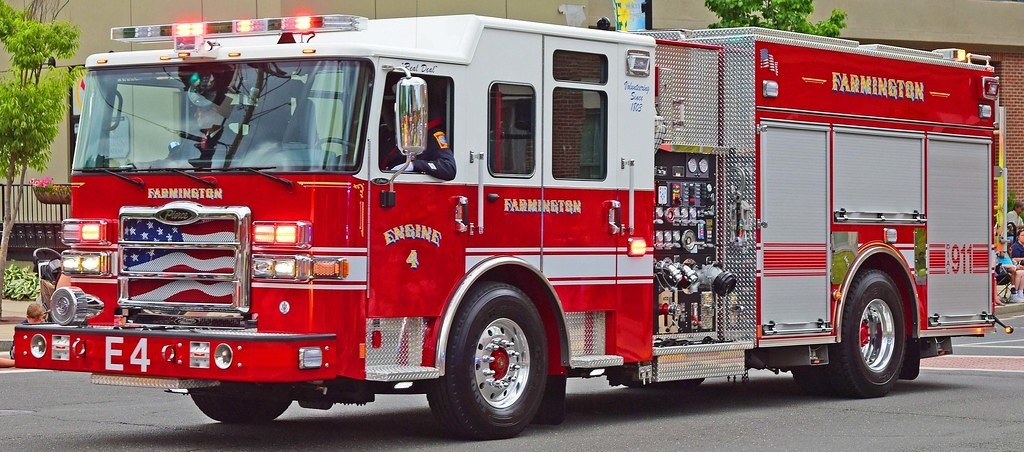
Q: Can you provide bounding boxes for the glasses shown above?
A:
[1019,229,1024,232]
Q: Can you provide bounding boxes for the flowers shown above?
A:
[27,177,71,196]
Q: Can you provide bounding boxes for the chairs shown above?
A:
[995,260,1022,303]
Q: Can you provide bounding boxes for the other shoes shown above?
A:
[1018,290,1024,299]
[995,301,1005,306]
[1009,294,1024,303]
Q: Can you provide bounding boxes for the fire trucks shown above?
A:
[7,12,1015,439]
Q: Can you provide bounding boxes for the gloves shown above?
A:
[390,162,414,171]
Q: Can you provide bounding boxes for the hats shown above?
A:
[368,72,403,100]
[1014,202,1024,210]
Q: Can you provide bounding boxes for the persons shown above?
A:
[995,202,1024,306]
[165,93,249,163]
[0,358,14,367]
[379,84,457,181]
[10,303,44,359]
